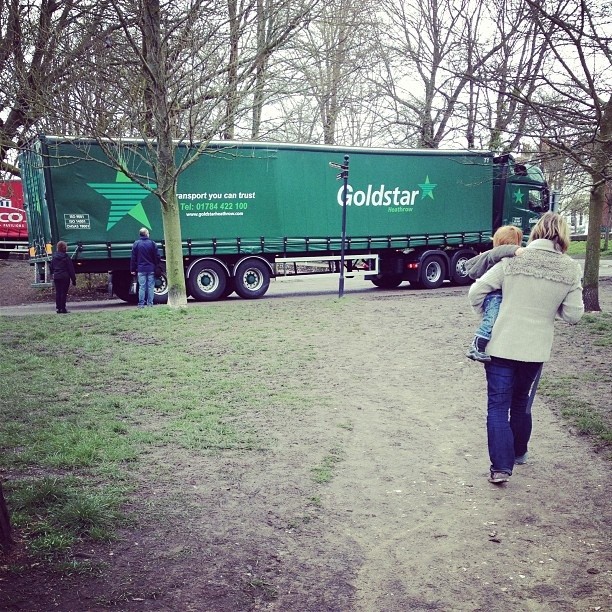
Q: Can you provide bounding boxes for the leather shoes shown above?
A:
[56,309,60,313]
[60,309,70,313]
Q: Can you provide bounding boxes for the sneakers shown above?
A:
[488,471,510,483]
[466,337,491,362]
[514,457,527,465]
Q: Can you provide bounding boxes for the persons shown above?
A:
[465,225,526,362]
[468,211,584,484]
[52,241,76,314]
[130,227,162,308]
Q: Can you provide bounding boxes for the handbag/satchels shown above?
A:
[152,241,162,278]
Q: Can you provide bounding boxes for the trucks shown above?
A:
[17,135,553,299]
